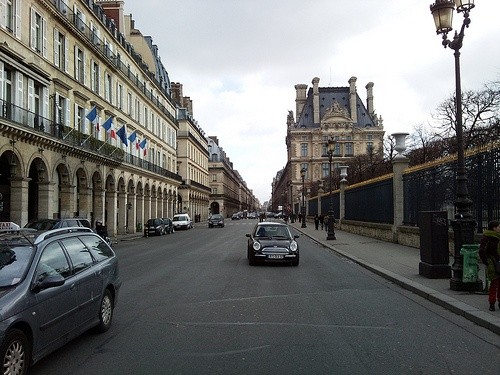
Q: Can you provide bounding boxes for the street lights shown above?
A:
[299,168,308,228]
[290,182,295,223]
[429,0,484,292]
[325,137,337,241]
[281,190,287,219]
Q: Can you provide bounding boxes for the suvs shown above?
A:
[172,213,191,229]
[0,225,122,375]
[23,219,92,234]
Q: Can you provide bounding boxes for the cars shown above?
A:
[0,221,37,241]
[144,218,166,236]
[275,212,285,219]
[207,214,224,228]
[162,218,174,234]
[245,222,300,266]
[231,211,274,220]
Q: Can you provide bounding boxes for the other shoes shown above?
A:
[489,302,496,311]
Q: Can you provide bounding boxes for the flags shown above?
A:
[102,116,116,139]
[86,107,99,131]
[139,139,146,156]
[128,131,139,149]
[116,125,128,147]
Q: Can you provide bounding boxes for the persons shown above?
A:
[320,213,328,231]
[260,213,266,222]
[314,214,320,230]
[199,214,201,222]
[479,220,500,312]
[286,210,302,223]
[96,221,104,238]
